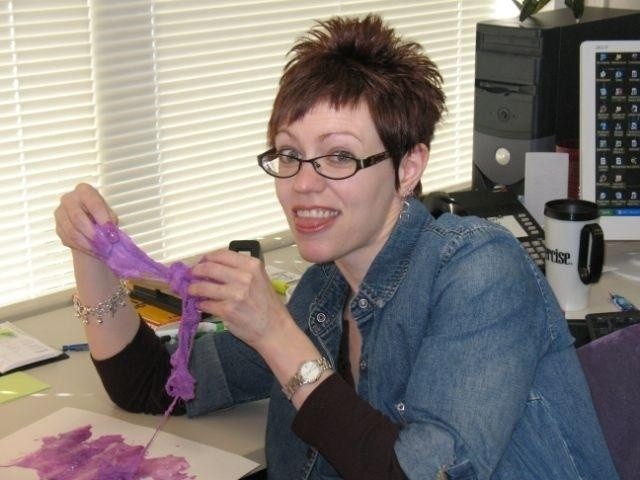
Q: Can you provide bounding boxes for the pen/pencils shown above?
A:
[62,344,89,351]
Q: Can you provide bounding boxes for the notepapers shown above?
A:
[0,370,52,405]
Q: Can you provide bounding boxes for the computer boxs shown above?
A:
[472,5,640,198]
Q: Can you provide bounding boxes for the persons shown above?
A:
[51,10,617,478]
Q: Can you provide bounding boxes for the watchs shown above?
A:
[281,356,332,401]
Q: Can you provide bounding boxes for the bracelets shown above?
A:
[71,281,131,327]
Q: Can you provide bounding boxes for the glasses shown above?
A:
[256,146,388,181]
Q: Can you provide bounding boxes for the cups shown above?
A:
[542,198,605,312]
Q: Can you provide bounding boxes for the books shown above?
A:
[0,321,69,378]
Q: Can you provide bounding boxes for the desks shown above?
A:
[0,182,640,480]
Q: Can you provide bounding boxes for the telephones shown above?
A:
[422,189,546,277]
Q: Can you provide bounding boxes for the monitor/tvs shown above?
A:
[579,39,640,240]
